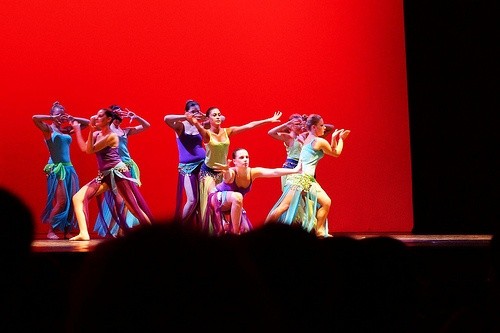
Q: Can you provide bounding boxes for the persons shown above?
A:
[33,102,90,240]
[185,107,283,236]
[0,189,500,333]
[69,110,151,241]
[93,105,150,240]
[264,114,351,237]
[164,100,225,229]
[202,148,302,234]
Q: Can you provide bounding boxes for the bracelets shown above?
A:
[339,136,344,140]
